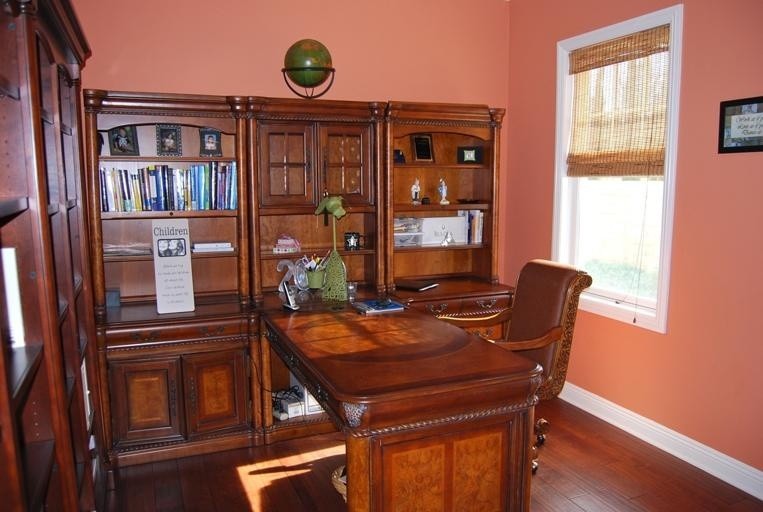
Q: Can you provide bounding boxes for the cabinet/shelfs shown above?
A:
[246,96,387,204]
[246,205,387,307]
[387,100,506,291]
[392,291,514,342]
[0,1,115,512]
[83,89,245,314]
[93,316,250,465]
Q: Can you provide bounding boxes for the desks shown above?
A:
[258,302,544,511]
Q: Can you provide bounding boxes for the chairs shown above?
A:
[433,257,593,472]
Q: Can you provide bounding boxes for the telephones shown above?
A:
[282,281,300,310]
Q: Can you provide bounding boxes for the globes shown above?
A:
[282,39,336,99]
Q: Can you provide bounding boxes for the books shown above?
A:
[100,161,238,212]
[458,209,484,244]
[190,242,235,253]
[353,299,405,313]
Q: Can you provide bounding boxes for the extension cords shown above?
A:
[272,405,289,421]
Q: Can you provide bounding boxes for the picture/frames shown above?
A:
[199,127,222,157]
[154,124,182,157]
[107,124,139,157]
[410,133,434,163]
[716,95,763,154]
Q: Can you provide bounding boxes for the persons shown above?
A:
[160,133,175,151]
[438,179,447,202]
[204,136,217,150]
[169,240,183,256]
[158,240,172,257]
[410,180,420,204]
[114,127,134,153]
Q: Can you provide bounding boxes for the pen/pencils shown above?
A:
[303,248,332,271]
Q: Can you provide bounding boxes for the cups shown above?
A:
[306,270,326,290]
[347,281,358,302]
[344,231,366,250]
